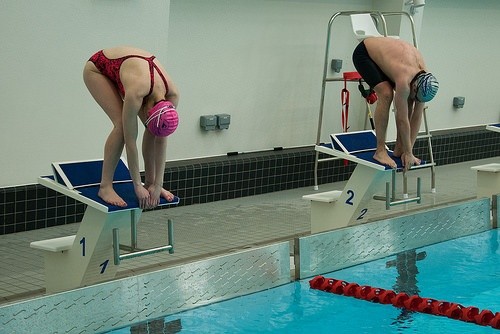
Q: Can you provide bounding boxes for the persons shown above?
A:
[83,45,179,210]
[352,36,439,175]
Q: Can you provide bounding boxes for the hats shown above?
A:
[146,101,179,137]
[414,72,439,102]
